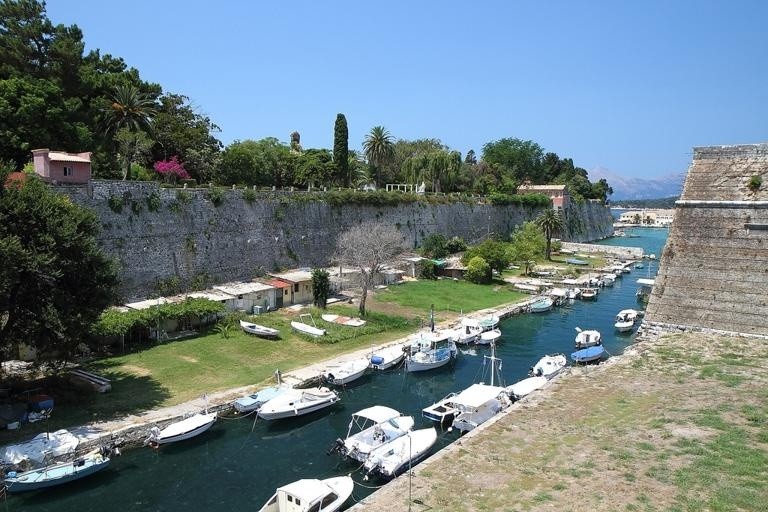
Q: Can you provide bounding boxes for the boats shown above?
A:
[549,288,570,308]
[0,438,125,496]
[406,346,452,372]
[614,317,634,333]
[420,383,513,432]
[142,410,218,446]
[587,259,644,287]
[616,308,638,323]
[529,297,553,313]
[326,415,415,463]
[454,324,484,344]
[239,320,280,338]
[568,287,584,300]
[527,352,568,380]
[320,357,369,385]
[364,426,438,478]
[368,343,405,370]
[233,383,296,413]
[474,328,502,344]
[257,385,341,421]
[636,278,656,288]
[290,320,327,338]
[581,287,597,301]
[570,344,605,363]
[574,329,601,348]
[504,375,548,400]
[478,314,500,332]
[261,472,355,509]
[321,313,366,328]
